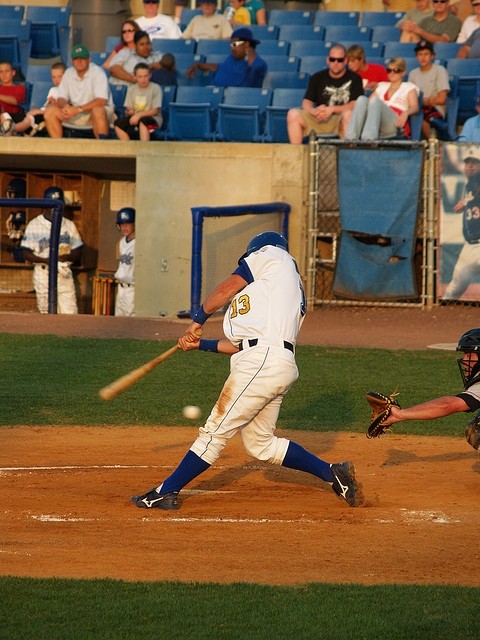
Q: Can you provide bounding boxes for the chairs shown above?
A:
[0,5,127,120]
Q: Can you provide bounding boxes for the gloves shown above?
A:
[57,261,72,278]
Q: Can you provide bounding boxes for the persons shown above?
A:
[114,63,163,141]
[407,40,451,141]
[457,0,480,43]
[131,231,363,510]
[113,208,135,318]
[224,0,251,26]
[345,56,421,141]
[227,0,267,26]
[376,328,480,451]
[180,0,234,40]
[0,62,67,137]
[187,28,267,87]
[402,1,461,43]
[20,186,84,314]
[43,44,115,139]
[0,62,26,137]
[457,98,480,143]
[443,145,480,300]
[108,30,163,88]
[394,0,457,43]
[134,0,183,39]
[346,45,390,90]
[150,54,177,84]
[100,20,141,68]
[287,44,365,147]
[455,27,480,59]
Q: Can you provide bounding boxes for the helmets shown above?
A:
[44,186,64,199]
[456,328,480,391]
[5,178,26,198]
[12,211,26,224]
[238,231,288,265]
[116,207,135,224]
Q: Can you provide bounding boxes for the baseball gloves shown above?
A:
[365,392,401,438]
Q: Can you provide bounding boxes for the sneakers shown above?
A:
[131,487,179,510]
[330,461,356,508]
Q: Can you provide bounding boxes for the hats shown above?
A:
[231,27,260,44]
[414,40,433,52]
[462,145,480,162]
[71,44,89,59]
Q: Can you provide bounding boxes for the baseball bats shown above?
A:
[98,327,202,403]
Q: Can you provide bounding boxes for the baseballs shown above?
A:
[183,405,200,421]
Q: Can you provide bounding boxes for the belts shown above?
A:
[239,338,293,353]
[121,282,130,287]
[469,239,480,244]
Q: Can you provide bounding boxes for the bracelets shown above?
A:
[193,305,212,326]
[198,339,220,354]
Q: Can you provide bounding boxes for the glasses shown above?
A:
[387,67,403,73]
[473,3,480,6]
[145,1,157,3]
[203,1,215,4]
[433,1,446,3]
[122,29,135,33]
[230,41,245,47]
[329,57,344,62]
